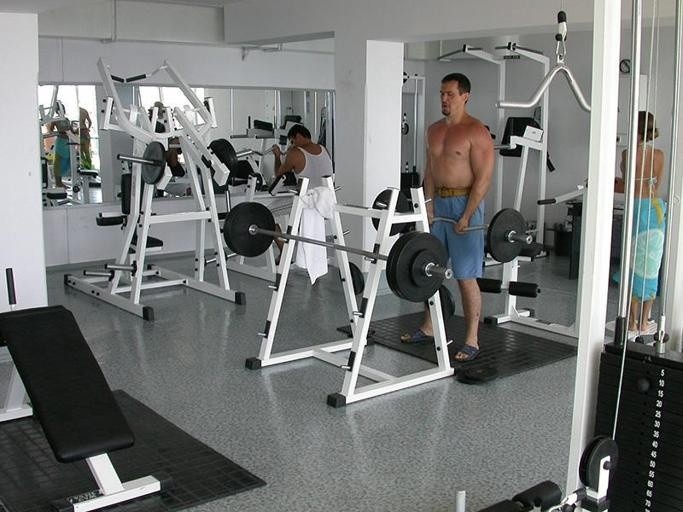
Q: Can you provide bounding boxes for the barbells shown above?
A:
[224,202,454,302]
[372,189,532,262]
[116,138,254,184]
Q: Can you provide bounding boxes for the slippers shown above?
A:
[455,344,480,362]
[402,329,434,343]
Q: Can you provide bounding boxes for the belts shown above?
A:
[436,187,470,197]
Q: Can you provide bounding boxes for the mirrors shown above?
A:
[400,93,418,191]
[41,80,335,210]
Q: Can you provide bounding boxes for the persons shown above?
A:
[614,111,665,332]
[79,107,92,168]
[400,72,494,361]
[48,102,73,190]
[43,120,57,155]
[147,102,185,178]
[272,124,335,265]
